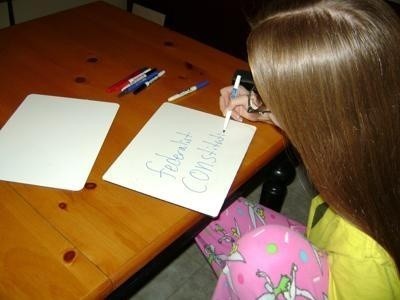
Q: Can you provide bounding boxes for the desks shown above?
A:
[0,0,315,299]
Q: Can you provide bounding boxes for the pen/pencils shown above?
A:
[106,66,167,98]
[167,79,209,102]
[222,75,242,133]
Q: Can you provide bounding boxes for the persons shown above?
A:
[195,0,400,300]
